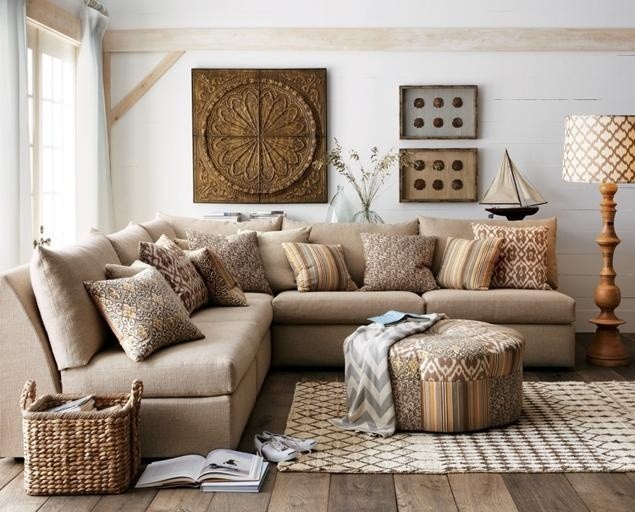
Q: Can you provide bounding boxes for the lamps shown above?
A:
[562,114,635,367]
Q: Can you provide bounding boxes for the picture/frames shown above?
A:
[399,85,478,140]
[399,148,478,203]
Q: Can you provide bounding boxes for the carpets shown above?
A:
[276,379,635,474]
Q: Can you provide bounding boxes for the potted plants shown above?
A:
[312,137,422,224]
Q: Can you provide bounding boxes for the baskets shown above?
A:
[20,377,144,498]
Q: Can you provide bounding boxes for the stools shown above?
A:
[357,318,526,434]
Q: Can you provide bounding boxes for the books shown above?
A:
[367,308,430,327]
[134,448,265,485]
[199,459,272,494]
[48,394,122,414]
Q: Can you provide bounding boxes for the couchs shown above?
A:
[1,265,574,464]
